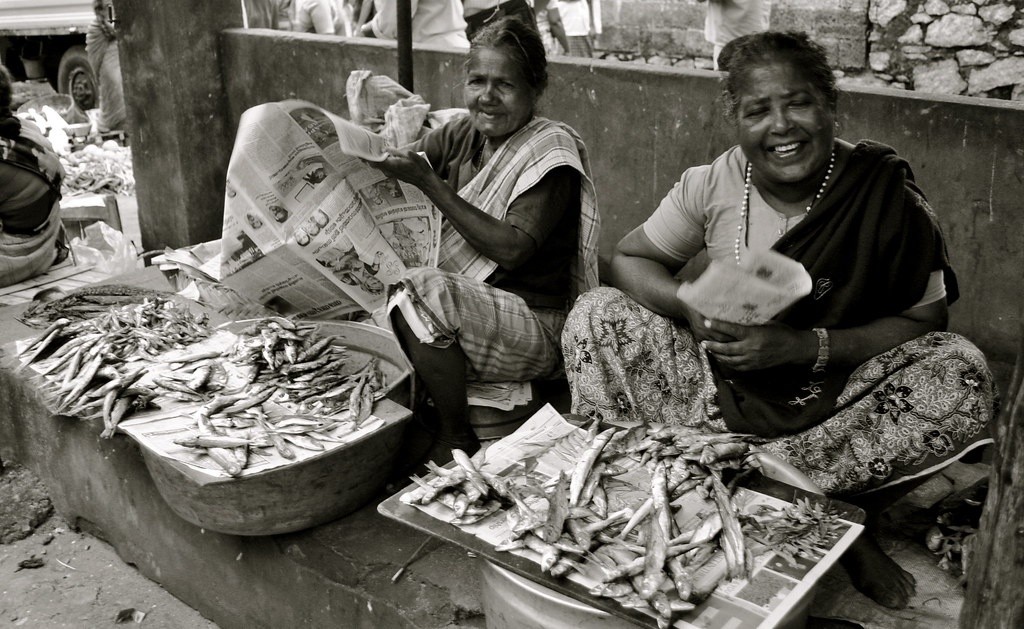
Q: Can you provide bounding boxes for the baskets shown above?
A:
[17,93,75,123]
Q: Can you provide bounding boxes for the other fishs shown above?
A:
[410,407,784,629]
[52,142,136,198]
[17,298,389,477]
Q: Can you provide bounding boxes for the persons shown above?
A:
[367,14,601,472]
[246,213,263,229]
[316,234,385,295]
[294,211,329,246]
[268,205,288,223]
[360,178,403,205]
[86,0,126,132]
[0,61,67,287]
[561,32,1000,607]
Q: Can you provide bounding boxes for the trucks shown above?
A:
[0,2,101,113]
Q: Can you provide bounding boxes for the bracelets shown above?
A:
[812,328,831,373]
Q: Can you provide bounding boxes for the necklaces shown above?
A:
[734,147,835,267]
[476,140,488,172]
